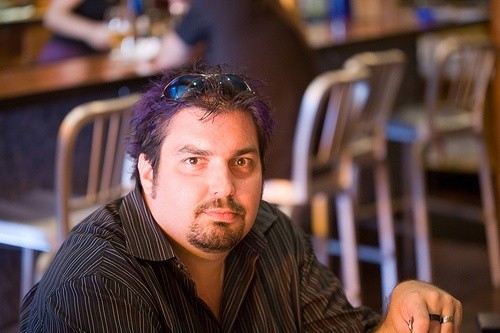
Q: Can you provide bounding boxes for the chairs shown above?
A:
[0,92,145,312]
[260,47,406,313]
[368,34,500,287]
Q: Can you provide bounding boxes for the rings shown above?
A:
[442,315,454,323]
[429,313,443,324]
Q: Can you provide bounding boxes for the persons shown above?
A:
[18,58,463,333]
[34,0,329,181]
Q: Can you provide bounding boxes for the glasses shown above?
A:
[159,73,256,104]
